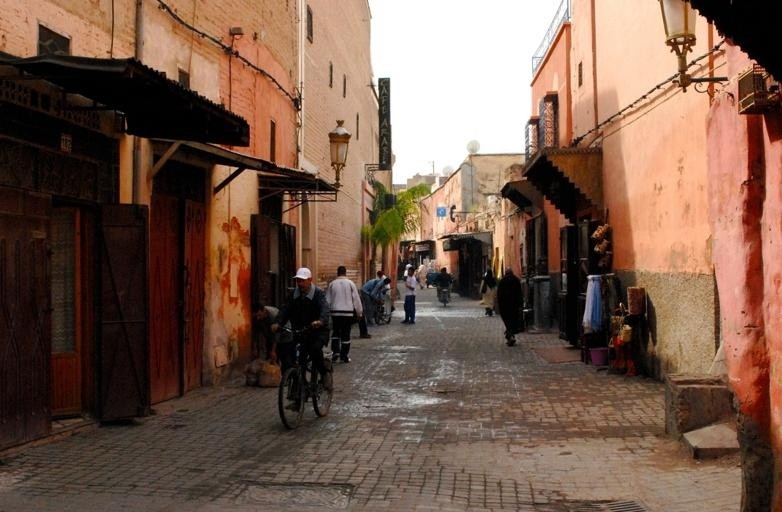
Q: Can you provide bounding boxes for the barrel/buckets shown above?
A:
[590,347,608,366]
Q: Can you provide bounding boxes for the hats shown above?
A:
[292,268,312,280]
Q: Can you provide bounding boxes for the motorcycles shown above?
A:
[432,279,453,307]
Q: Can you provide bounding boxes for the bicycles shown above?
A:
[275,324,334,429]
[373,286,392,326]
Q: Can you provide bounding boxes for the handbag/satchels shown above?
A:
[244,359,282,387]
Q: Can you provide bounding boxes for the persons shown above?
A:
[479,268,497,317]
[271,268,330,410]
[377,271,391,316]
[434,268,452,300]
[401,268,417,324]
[325,266,364,363]
[403,260,412,277]
[251,301,297,386]
[361,278,391,327]
[497,267,525,345]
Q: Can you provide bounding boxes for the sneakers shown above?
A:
[321,375,331,391]
[360,333,371,338]
[400,320,416,324]
[506,335,517,346]
[332,353,351,363]
[285,402,305,412]
